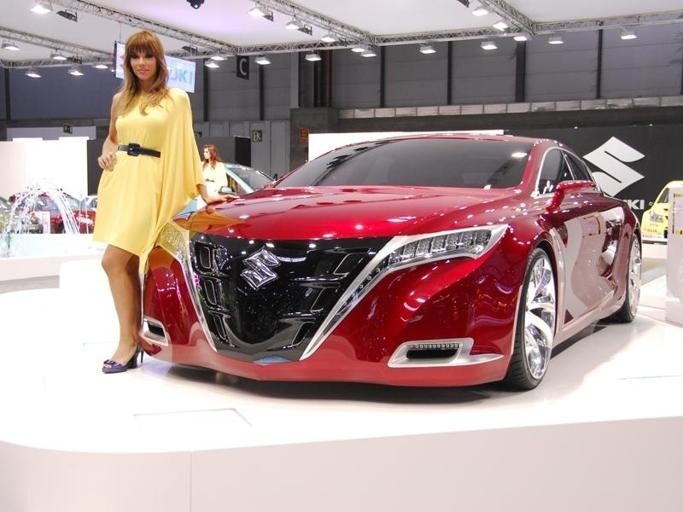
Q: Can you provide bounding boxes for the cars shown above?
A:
[637,179,683,243]
[0,187,99,233]
[221,161,277,200]
[131,126,645,396]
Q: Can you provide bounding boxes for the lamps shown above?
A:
[418,45,437,55]
[28,2,52,15]
[620,28,638,41]
[350,45,377,59]
[492,21,509,33]
[547,34,565,46]
[285,20,313,39]
[2,42,21,54]
[56,9,78,24]
[92,63,109,72]
[68,67,84,77]
[49,54,69,62]
[304,53,322,62]
[320,34,340,44]
[479,40,498,52]
[25,72,41,77]
[254,55,271,66]
[203,54,225,70]
[471,6,490,17]
[511,34,529,42]
[247,6,274,25]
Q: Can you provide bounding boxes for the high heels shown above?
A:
[102,344,144,373]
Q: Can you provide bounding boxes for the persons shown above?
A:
[195,145,228,208]
[89,31,236,374]
